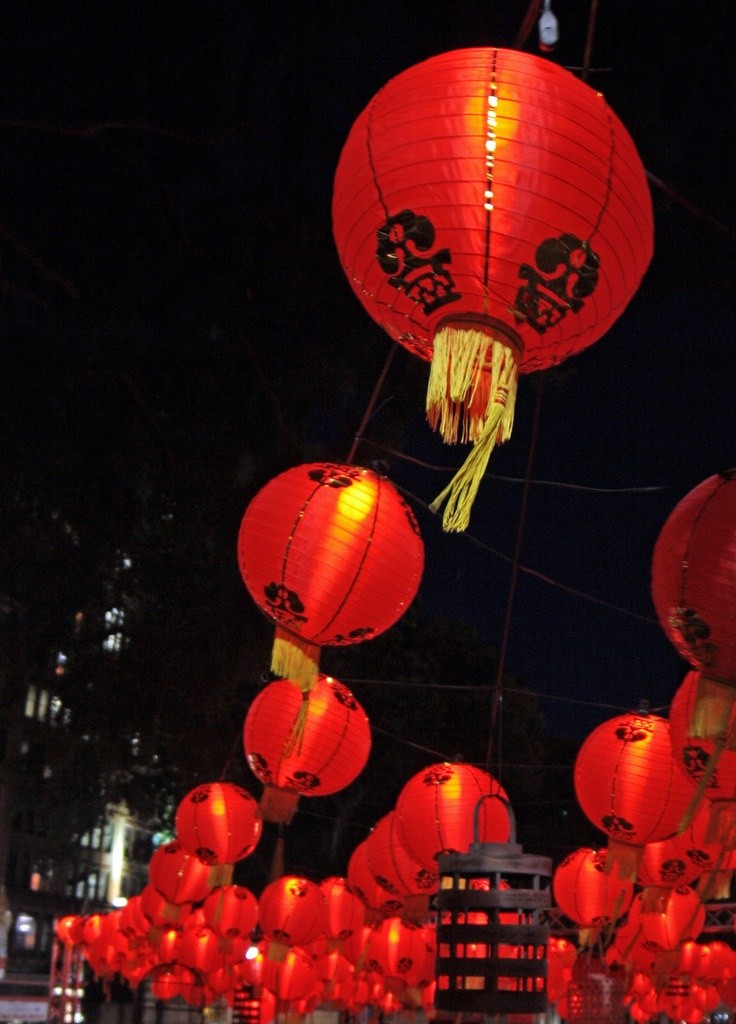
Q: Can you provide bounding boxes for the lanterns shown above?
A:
[234,462,425,757]
[242,672,373,823]
[396,761,513,877]
[368,809,439,925]
[652,467,735,836]
[175,782,262,927]
[572,712,707,882]
[669,672,736,850]
[333,46,654,531]
[59,841,734,1024]
